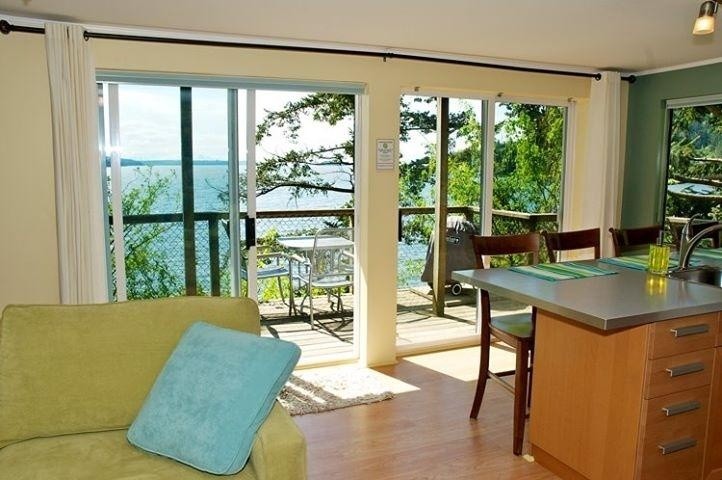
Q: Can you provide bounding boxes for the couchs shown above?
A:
[1,298,308,480]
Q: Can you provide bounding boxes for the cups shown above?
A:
[648,244,670,275]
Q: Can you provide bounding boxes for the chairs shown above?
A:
[469,231,542,454]
[542,229,600,263]
[610,226,666,257]
[221,216,354,331]
[669,220,720,251]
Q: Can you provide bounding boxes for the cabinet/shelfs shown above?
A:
[637,311,721,480]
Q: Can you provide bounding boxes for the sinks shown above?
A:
[670,265,722,287]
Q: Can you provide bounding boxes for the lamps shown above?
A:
[691,1,721,36]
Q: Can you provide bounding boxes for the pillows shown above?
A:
[125,321,302,476]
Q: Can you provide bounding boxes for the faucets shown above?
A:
[678,213,722,269]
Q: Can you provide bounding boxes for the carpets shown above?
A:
[275,363,395,415]
